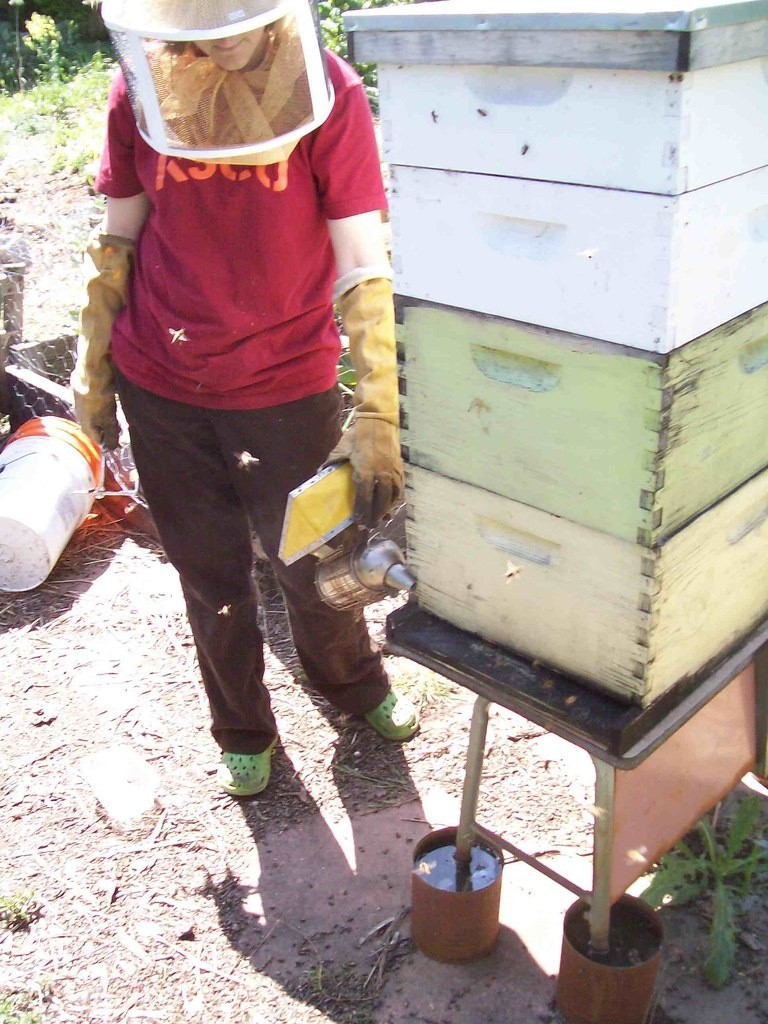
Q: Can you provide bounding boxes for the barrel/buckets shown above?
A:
[0,416,102,592]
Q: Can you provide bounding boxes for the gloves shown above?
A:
[316,265,406,530]
[72,233,136,452]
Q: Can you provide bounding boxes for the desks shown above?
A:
[387,603,768,954]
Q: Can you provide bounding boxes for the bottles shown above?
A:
[312,529,418,612]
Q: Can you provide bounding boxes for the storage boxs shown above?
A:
[4,336,164,544]
[365,63,767,710]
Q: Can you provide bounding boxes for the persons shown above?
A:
[72,1,420,798]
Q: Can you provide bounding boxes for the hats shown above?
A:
[100,0,337,157]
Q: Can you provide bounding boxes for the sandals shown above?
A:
[364,684,417,741]
[215,732,279,795]
